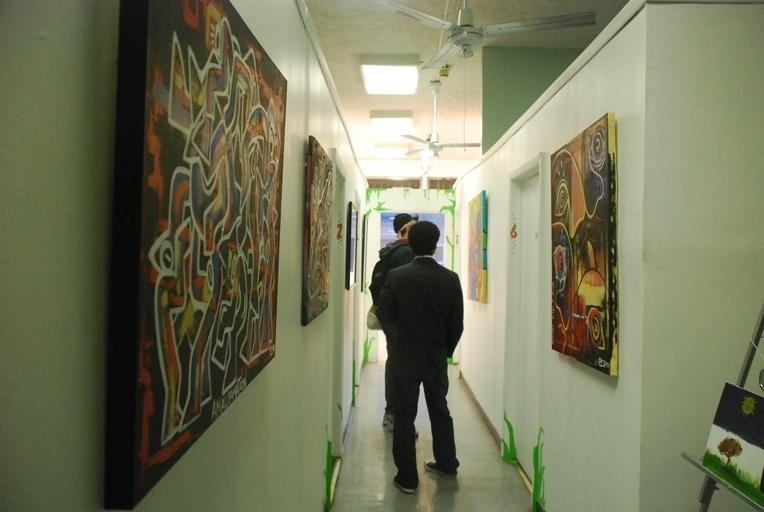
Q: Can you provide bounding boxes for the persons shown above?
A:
[368,213,421,440]
[377,220,466,495]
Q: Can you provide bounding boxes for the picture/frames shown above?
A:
[346,201,358,291]
[361,214,368,292]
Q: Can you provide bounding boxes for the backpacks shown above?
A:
[369,243,409,306]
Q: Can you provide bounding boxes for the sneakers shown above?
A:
[394,475,417,494]
[383,411,418,438]
[424,461,458,479]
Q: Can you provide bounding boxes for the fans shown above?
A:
[401,84,482,155]
[387,0,596,69]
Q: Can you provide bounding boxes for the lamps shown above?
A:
[369,110,413,127]
[358,53,420,95]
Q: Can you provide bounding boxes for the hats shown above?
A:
[393,213,417,233]
